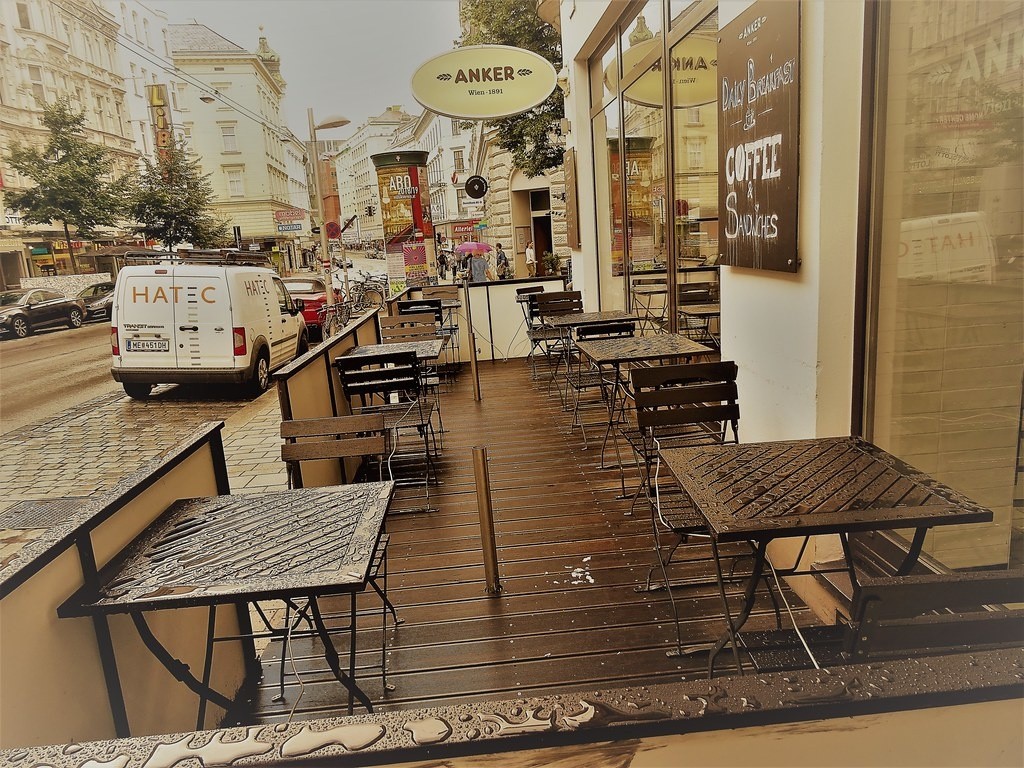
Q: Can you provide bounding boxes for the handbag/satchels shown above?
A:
[467,270,473,282]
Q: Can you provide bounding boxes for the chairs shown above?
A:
[630,278,721,351]
[280,287,458,690]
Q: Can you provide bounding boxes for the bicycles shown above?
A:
[315,270,389,340]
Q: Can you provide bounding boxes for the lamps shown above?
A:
[561,118,572,137]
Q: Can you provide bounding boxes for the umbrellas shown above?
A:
[458,242,491,253]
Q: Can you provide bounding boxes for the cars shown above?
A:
[77,281,116,322]
[339,257,353,269]
[281,277,346,345]
[0,288,88,339]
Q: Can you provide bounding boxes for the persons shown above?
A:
[495,243,509,281]
[524,241,538,277]
[339,241,384,250]
[436,249,491,282]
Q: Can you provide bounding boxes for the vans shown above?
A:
[109,263,310,402]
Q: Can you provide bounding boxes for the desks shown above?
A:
[57,481,395,712]
[516,294,536,379]
[545,310,639,412]
[678,304,720,348]
[657,436,994,679]
[517,286,1024,675]
[577,333,715,499]
[632,284,667,336]
[340,339,445,458]
[410,299,461,386]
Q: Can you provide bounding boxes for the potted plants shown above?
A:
[541,251,561,277]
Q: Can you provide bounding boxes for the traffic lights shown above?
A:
[364,205,376,216]
